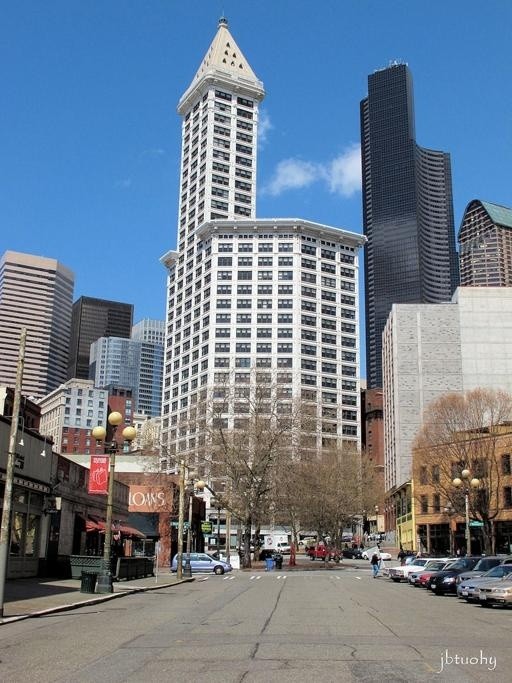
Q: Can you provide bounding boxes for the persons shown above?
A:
[371,553,381,578]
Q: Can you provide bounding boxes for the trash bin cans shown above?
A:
[80,569,99,592]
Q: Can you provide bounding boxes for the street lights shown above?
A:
[92,411,137,593]
[452,468,480,556]
[211,494,228,562]
[184,470,206,577]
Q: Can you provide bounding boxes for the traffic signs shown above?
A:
[173,522,189,529]
[468,520,484,527]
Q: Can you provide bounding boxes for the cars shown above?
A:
[343,548,364,560]
[340,535,353,542]
[275,542,292,554]
[383,550,512,607]
[171,552,232,575]
[307,544,343,562]
[298,537,316,545]
[362,548,391,560]
